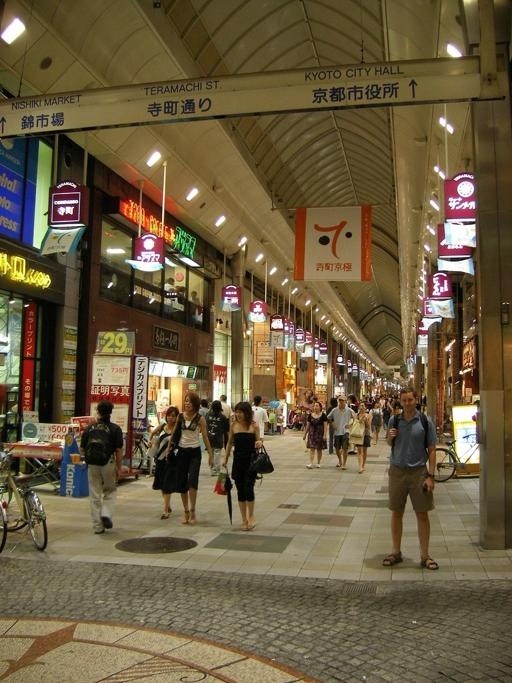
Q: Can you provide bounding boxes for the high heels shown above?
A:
[181,511,189,523]
[188,510,196,524]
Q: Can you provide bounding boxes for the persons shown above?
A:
[222,401,264,531]
[80,400,124,536]
[146,407,184,521]
[350,401,373,473]
[365,397,371,409]
[383,400,394,429]
[374,394,383,398]
[303,402,328,469]
[169,393,215,524]
[368,400,384,445]
[221,395,233,419]
[252,396,269,445]
[382,387,441,571]
[198,398,210,417]
[204,400,230,476]
[326,396,356,470]
[220,393,232,451]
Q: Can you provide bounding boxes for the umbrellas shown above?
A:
[223,463,234,526]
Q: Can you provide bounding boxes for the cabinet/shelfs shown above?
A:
[5,387,21,474]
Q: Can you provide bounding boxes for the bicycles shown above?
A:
[122,420,157,468]
[427,433,480,482]
[0,447,48,553]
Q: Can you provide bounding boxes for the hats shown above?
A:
[338,395,347,400]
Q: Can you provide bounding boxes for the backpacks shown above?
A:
[83,420,114,465]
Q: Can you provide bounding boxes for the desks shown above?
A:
[8,427,72,497]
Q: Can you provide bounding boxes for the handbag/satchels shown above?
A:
[351,419,365,437]
[350,436,364,444]
[173,413,183,447]
[249,443,274,474]
[146,423,166,461]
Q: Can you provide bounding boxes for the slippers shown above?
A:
[342,467,347,469]
[336,463,342,467]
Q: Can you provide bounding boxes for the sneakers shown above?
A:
[316,464,320,467]
[95,529,104,534]
[101,515,112,529]
[306,464,313,468]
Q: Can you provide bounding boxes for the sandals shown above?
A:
[242,520,248,531]
[168,508,172,513]
[383,551,403,565]
[248,517,256,530]
[421,557,439,570]
[160,512,169,519]
